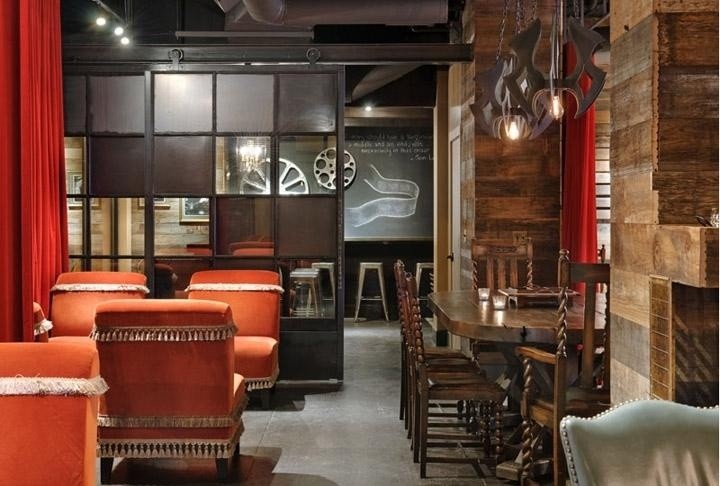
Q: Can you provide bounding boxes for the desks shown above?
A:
[426,288,605,481]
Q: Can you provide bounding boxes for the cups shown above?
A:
[492,295,506,309]
[478,287,491,301]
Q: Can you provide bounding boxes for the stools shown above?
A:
[290,261,433,322]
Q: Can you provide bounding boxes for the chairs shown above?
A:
[174,240,276,268]
[1,341,110,486]
[469,236,610,486]
[394,258,507,479]
[140,262,177,300]
[559,396,718,486]
[89,300,250,486]
[183,270,285,409]
[33,271,150,342]
